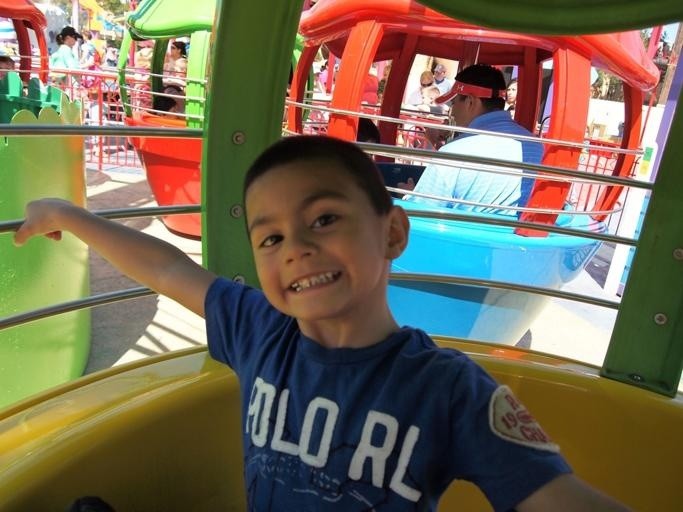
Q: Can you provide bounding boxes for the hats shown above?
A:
[61,27,83,41]
[434,78,507,105]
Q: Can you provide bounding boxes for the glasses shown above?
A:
[72,35,78,40]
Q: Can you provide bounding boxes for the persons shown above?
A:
[103,45,117,66]
[13,135,633,512]
[0,56,15,80]
[407,59,544,217]
[49,26,101,97]
[357,117,380,157]
[162,42,187,119]
